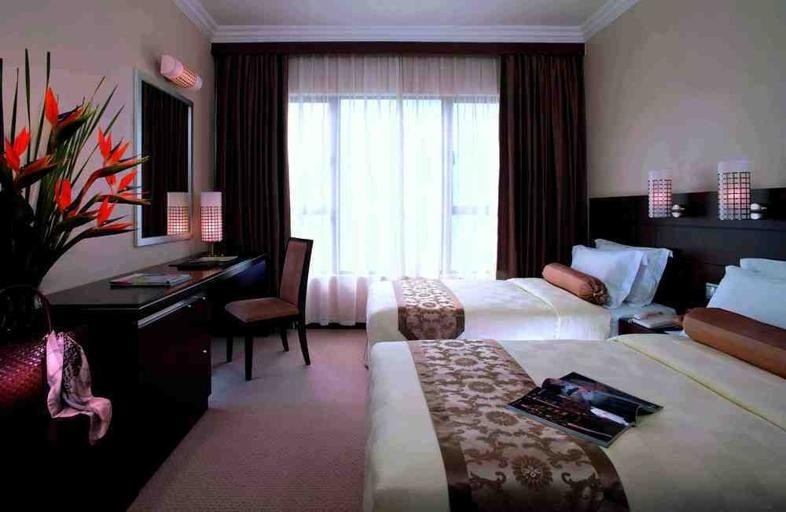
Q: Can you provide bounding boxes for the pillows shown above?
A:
[571,244,649,309]
[542,262,607,303]
[740,257,786,277]
[685,309,786,381]
[594,238,673,307]
[680,265,786,334]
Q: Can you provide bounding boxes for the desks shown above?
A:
[45,247,280,469]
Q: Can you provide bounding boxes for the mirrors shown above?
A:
[133,67,196,245]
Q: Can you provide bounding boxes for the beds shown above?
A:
[366,278,669,347]
[365,332,786,508]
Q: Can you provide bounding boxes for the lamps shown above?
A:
[159,53,204,93]
[645,169,675,219]
[164,191,190,237]
[715,161,753,218]
[200,191,225,255]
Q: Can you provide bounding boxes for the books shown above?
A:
[506,372,663,448]
[107,272,192,287]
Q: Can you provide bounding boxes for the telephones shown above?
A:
[633,304,677,329]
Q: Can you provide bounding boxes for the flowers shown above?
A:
[1,46,153,334]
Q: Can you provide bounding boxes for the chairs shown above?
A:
[223,237,317,383]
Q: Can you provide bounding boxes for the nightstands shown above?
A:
[618,313,686,338]
